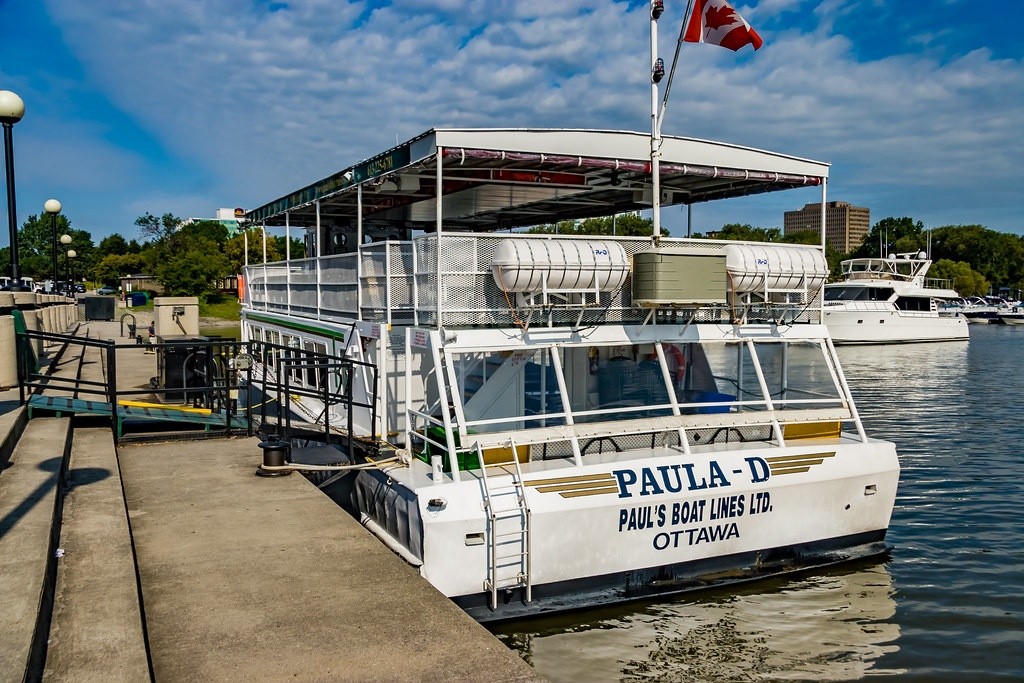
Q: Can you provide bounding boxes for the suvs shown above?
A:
[0,277,85,294]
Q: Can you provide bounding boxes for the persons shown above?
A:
[148,321,155,351]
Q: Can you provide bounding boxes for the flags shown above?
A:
[683,0,762,51]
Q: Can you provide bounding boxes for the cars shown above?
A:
[96,286,116,295]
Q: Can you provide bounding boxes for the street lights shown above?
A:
[127,274,131,294]
[45,199,63,306]
[68,250,76,299]
[60,235,73,305]
[0,91,32,316]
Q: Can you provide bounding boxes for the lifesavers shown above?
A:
[649,344,685,385]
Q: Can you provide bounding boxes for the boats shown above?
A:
[790,226,1024,345]
[222,0,899,627]
